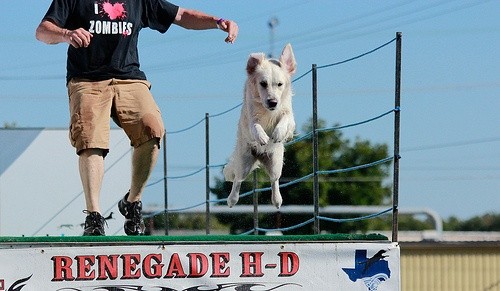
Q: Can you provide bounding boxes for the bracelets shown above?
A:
[217,18,224,29]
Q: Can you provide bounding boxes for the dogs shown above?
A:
[223,42,297,209]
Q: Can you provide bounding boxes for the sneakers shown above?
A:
[81,210,109,236]
[117,191,145,236]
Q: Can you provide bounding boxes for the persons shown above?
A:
[35,0,239,236]
